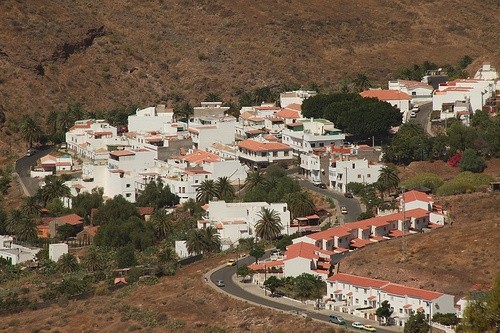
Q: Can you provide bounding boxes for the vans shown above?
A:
[27,149,34,157]
[227,259,236,267]
[328,315,346,325]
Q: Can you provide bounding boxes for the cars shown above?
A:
[313,177,353,216]
[217,280,225,287]
[364,325,376,332]
[352,322,365,329]
